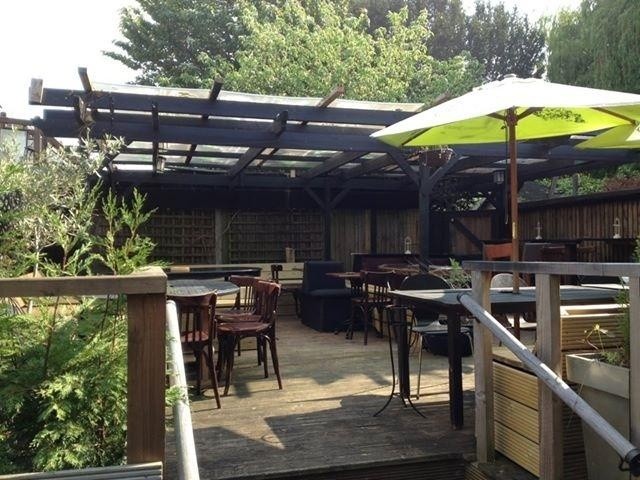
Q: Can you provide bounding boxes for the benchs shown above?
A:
[300,260,369,333]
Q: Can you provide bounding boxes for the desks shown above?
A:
[388,285,628,431]
[167,277,240,380]
[324,272,362,339]
[282,283,301,317]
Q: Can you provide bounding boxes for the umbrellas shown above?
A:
[368,73,640,344]
[572,122,640,149]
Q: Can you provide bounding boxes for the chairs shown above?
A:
[482,241,597,284]
[178,264,283,408]
[349,269,536,402]
